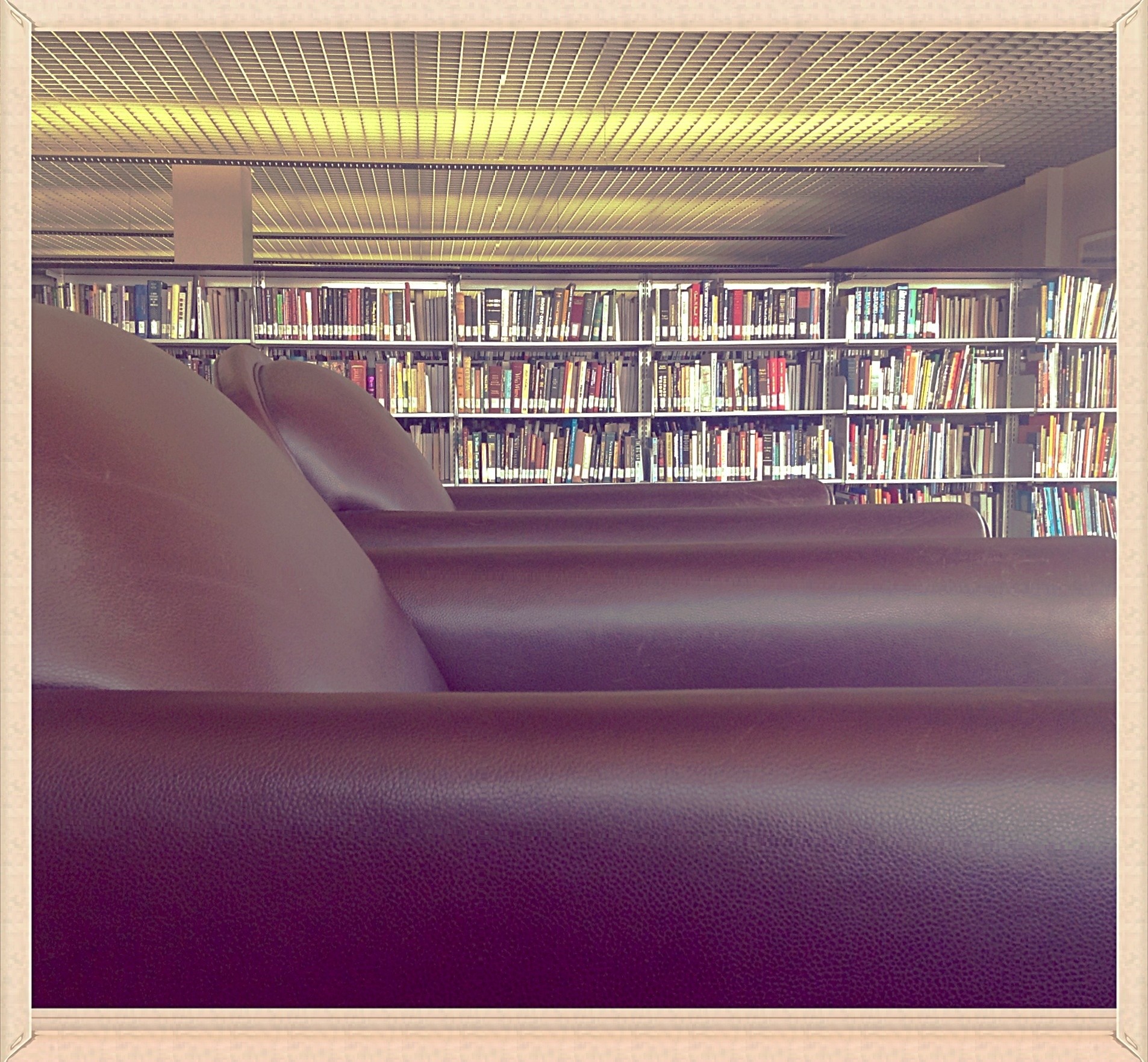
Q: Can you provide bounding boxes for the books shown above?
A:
[651,279,826,342]
[1015,485,1116,538]
[456,350,637,413]
[652,420,835,482]
[1027,276,1117,339]
[396,417,449,480]
[841,345,1004,408]
[654,351,822,412]
[844,282,1009,338]
[849,414,994,479]
[1017,412,1116,478]
[1020,344,1116,408]
[32,275,251,339]
[253,282,447,341]
[454,284,639,342]
[835,483,1003,539]
[458,419,644,483]
[268,348,448,413]
[158,346,227,384]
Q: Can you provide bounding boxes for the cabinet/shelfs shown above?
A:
[31,272,1116,538]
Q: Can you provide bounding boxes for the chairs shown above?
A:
[32,300,1118,1010]
[208,346,992,539]
